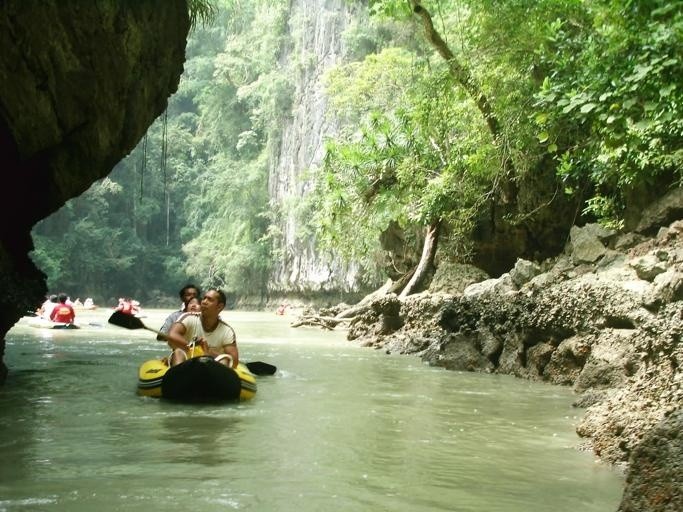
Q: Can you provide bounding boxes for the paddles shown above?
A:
[108,309,190,347]
[224,354,276,376]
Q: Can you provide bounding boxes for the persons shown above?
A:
[185,296,202,313]
[157,284,202,353]
[35,290,140,325]
[167,288,238,370]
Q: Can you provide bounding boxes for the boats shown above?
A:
[135,355,257,404]
[27,317,80,329]
